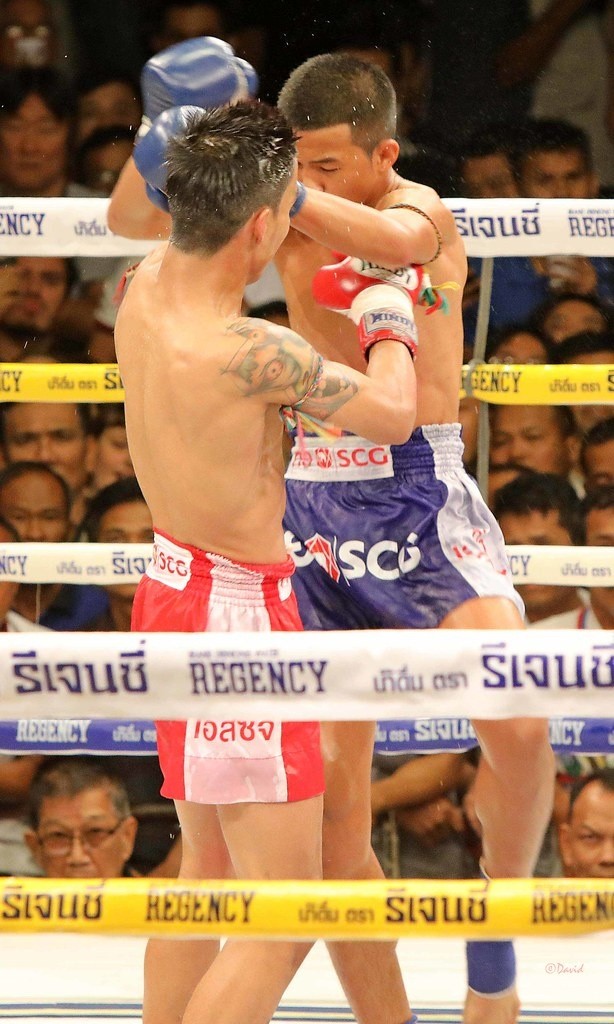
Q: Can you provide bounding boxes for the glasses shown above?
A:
[35,817,126,858]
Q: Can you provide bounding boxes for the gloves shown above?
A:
[136,36,254,148]
[313,249,423,364]
[131,105,304,217]
[114,263,141,306]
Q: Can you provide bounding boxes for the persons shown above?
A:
[105,35,554,1024]
[559,767,614,878]
[23,756,140,880]
[0,0,614,879]
[111,98,465,1024]
